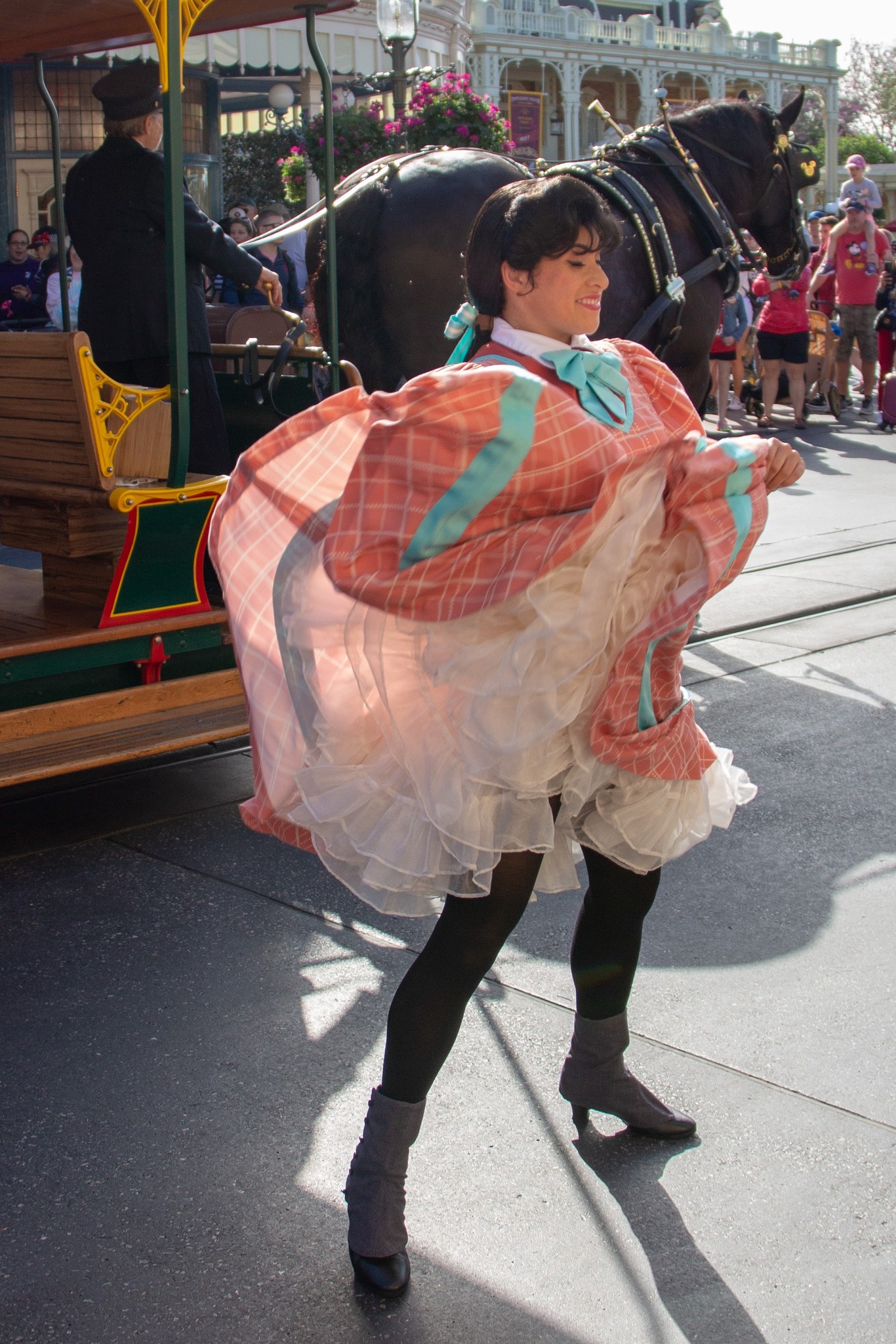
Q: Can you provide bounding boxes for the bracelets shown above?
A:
[778,281,782,289]
[25,293,29,297]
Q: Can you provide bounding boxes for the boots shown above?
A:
[344,1085,426,1295]
[558,1012,694,1138]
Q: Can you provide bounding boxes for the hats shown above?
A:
[232,196,257,209]
[845,201,866,213]
[94,65,166,120]
[229,207,246,219]
[844,154,866,168]
[268,203,289,215]
[807,211,825,221]
[26,226,58,249]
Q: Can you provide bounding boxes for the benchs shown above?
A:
[0,330,230,629]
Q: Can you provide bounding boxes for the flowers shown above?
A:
[276,70,516,204]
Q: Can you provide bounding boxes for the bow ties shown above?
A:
[541,349,633,434]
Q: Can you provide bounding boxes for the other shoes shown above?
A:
[846,398,853,408]
[758,416,774,426]
[794,417,807,428]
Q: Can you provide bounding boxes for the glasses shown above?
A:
[9,241,27,247]
[262,222,282,227]
[243,207,254,214]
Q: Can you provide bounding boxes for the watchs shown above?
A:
[24,294,31,302]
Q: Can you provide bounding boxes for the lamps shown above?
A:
[264,83,307,142]
[332,87,356,113]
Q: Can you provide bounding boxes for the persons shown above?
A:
[752,267,812,428]
[207,174,807,1298]
[206,197,256,304]
[709,290,747,431]
[238,209,303,319]
[803,203,877,408]
[708,230,768,409]
[45,240,83,332]
[264,203,308,293]
[0,227,71,322]
[816,154,882,276]
[65,59,283,472]
[875,271,896,423]
[806,200,894,414]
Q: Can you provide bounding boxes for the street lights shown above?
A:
[375,0,421,153]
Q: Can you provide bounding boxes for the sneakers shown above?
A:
[727,395,742,410]
[817,264,836,277]
[825,395,848,413]
[717,420,732,431]
[864,264,878,276]
[859,400,874,414]
[705,396,718,414]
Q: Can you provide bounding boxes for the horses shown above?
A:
[304,81,807,420]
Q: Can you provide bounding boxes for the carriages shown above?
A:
[1,0,818,807]
